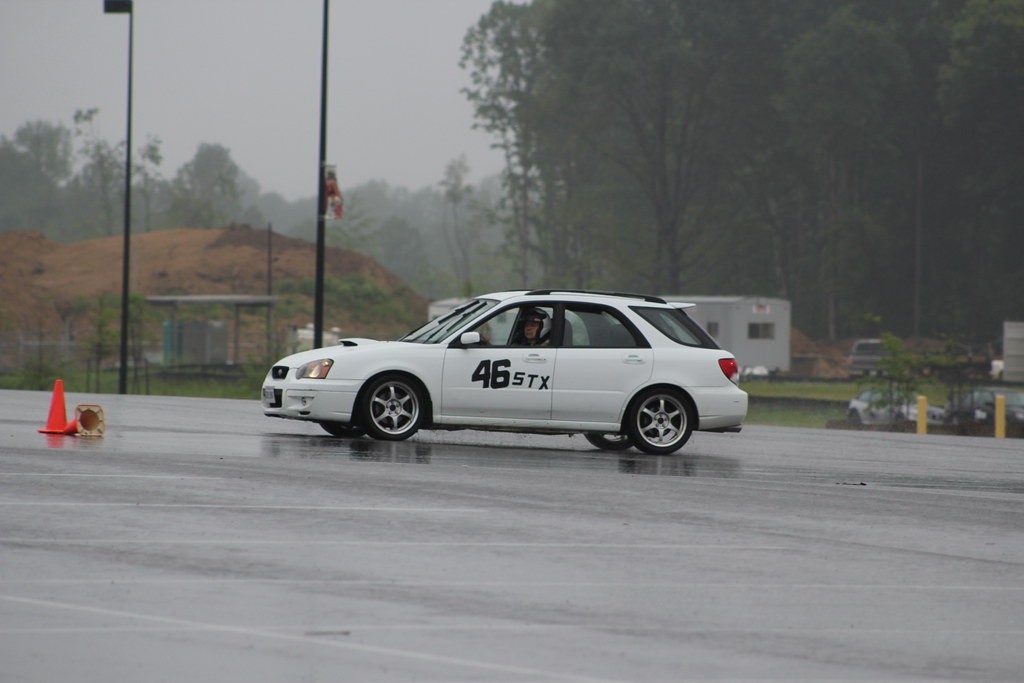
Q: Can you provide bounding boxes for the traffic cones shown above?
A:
[65,403,106,439]
[39,379,73,436]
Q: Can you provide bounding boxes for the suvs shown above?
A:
[260,286,751,456]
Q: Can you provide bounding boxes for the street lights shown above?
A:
[100,1,134,392]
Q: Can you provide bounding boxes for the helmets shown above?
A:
[521,308,551,343]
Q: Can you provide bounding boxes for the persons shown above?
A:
[480,308,552,347]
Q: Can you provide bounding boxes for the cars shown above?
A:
[840,336,892,379]
[844,386,946,428]
[944,386,1024,434]
